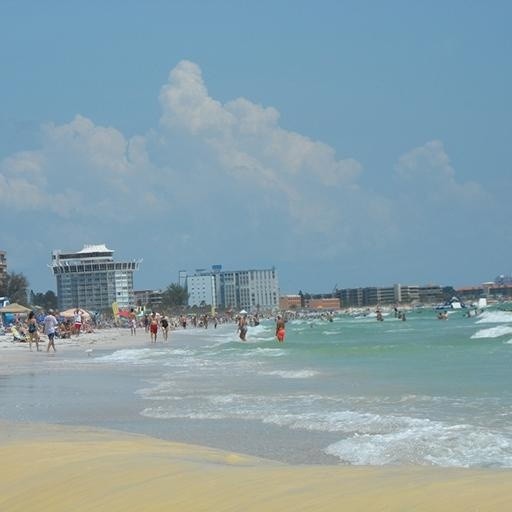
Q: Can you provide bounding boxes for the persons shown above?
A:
[0,306,482,353]
